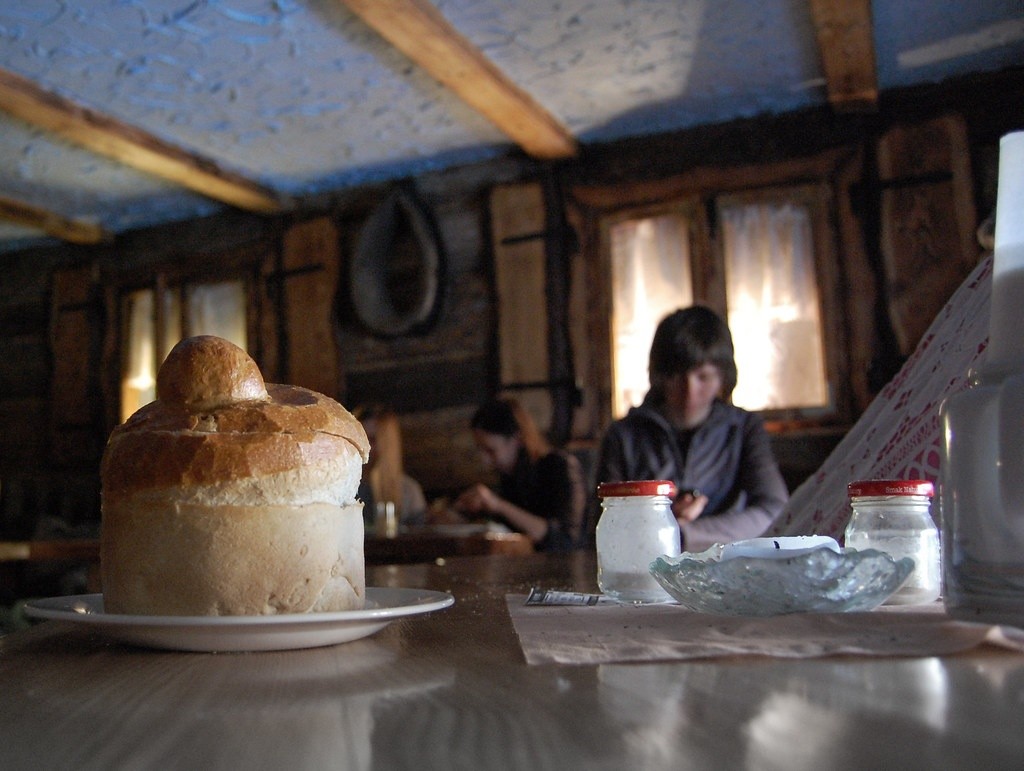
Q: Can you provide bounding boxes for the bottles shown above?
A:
[845,481,944,607]
[593,480,681,606]
[940,353,1024,627]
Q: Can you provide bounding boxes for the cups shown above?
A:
[375,503,399,539]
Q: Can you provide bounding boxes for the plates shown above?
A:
[399,524,487,540]
[22,584,455,652]
[651,542,917,613]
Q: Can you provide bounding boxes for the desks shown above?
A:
[0,553,1024,771]
[372,530,536,559]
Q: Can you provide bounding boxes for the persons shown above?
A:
[586,303,790,554]
[355,410,427,565]
[454,394,587,554]
[14,490,92,596]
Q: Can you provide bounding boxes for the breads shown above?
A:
[98,334,372,616]
